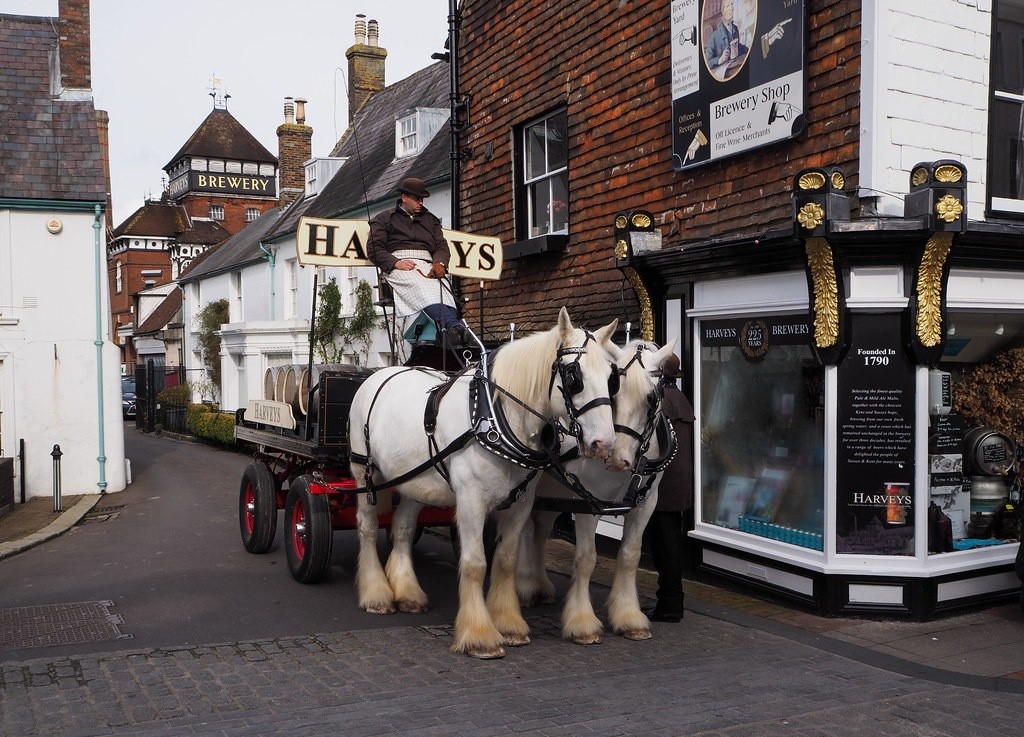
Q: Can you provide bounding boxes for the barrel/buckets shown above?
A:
[971,477,1008,511]
[963,427,1015,476]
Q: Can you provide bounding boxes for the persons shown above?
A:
[648,353,697,624]
[708,346,784,501]
[366,178,473,345]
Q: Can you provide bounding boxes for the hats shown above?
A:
[658,352,684,378]
[396,178,430,197]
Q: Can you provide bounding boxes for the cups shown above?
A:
[884,482,910,524]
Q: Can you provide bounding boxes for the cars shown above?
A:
[122,379,138,419]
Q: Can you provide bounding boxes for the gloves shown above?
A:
[428,261,446,278]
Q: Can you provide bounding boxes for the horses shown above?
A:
[345,304,678,670]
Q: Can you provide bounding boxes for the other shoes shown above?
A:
[448,325,470,346]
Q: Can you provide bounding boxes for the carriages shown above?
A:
[240,265,697,660]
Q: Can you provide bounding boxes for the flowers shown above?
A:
[546,199,565,221]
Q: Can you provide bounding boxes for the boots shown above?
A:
[641,596,684,623]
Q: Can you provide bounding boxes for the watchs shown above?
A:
[437,260,446,268]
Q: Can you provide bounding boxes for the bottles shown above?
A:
[928,501,954,552]
[968,512,991,539]
[999,501,1017,539]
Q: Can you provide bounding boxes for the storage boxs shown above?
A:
[928,413,964,455]
[930,473,963,494]
[931,491,972,529]
[928,454,962,473]
